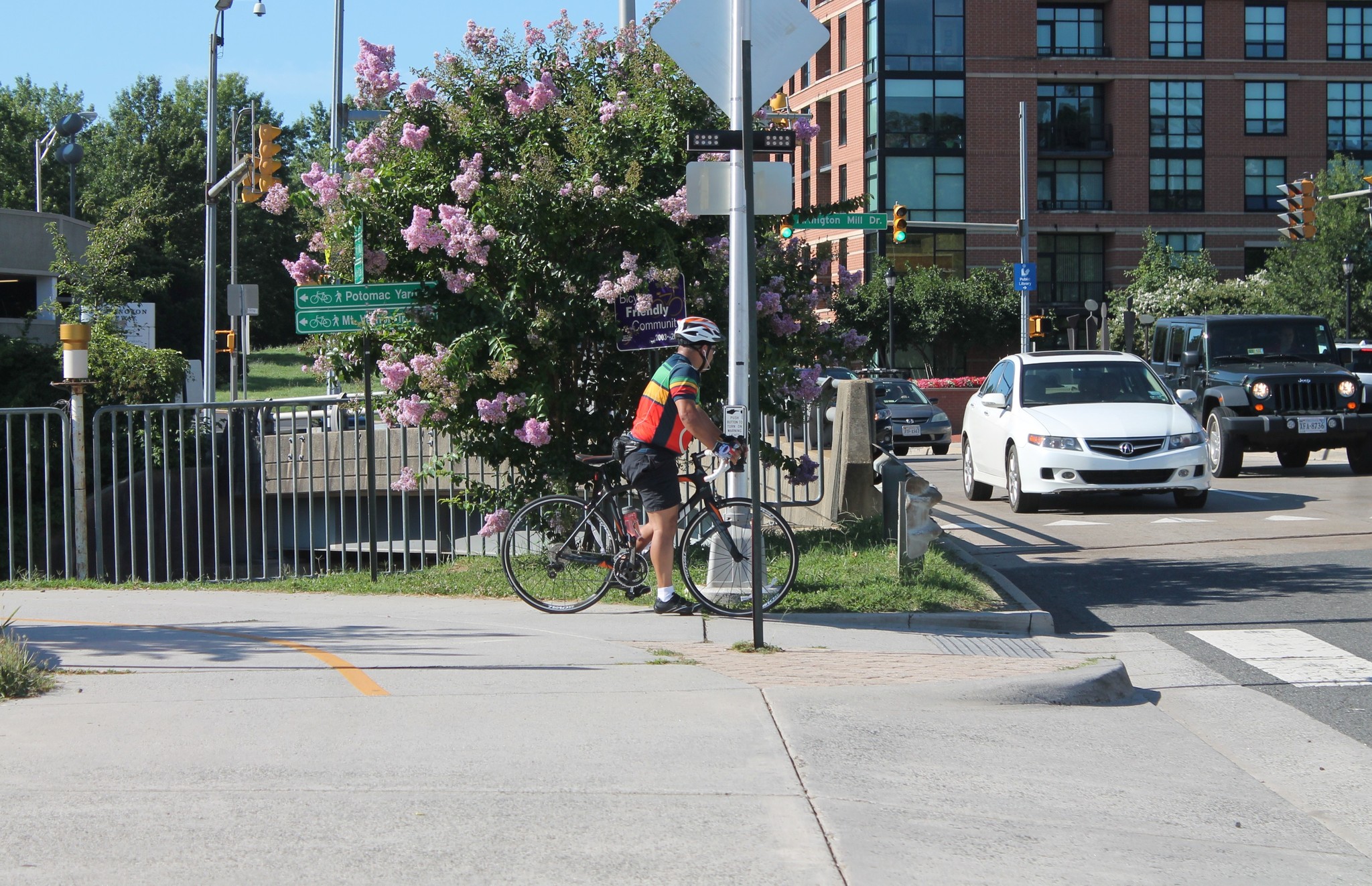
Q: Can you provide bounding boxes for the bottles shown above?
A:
[622,506,640,537]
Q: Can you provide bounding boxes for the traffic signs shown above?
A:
[296,306,439,334]
[296,280,439,308]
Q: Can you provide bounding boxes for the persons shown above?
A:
[1263,324,1303,353]
[621,316,748,614]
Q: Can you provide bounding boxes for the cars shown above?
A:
[861,377,953,455]
[961,349,1212,515]
[771,367,893,453]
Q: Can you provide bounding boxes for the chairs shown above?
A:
[1077,371,1103,398]
[1025,375,1047,401]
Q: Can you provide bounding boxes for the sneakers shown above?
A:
[654,592,703,614]
[604,573,651,594]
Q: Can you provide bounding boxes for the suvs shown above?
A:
[1149,314,1372,477]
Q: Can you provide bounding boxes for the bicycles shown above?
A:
[501,433,799,615]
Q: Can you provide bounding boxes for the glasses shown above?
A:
[708,345,719,354]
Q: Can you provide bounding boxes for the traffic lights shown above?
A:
[1274,178,1318,241]
[776,213,795,239]
[240,124,282,203]
[892,205,908,243]
[1363,175,1372,226]
[1035,318,1053,333]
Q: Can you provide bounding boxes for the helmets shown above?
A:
[674,317,726,346]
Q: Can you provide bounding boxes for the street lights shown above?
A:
[881,266,897,367]
[1342,253,1356,340]
[35,108,99,214]
